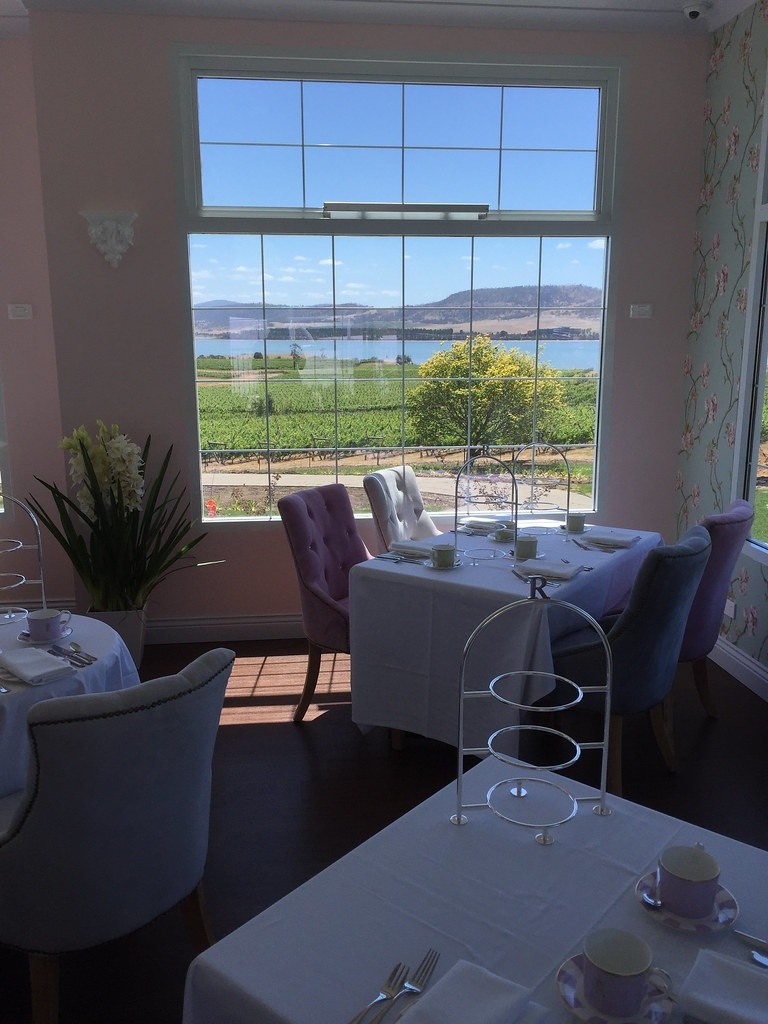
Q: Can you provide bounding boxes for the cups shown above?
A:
[585,929,672,1017]
[26,609,70,641]
[431,544,455,567]
[658,841,720,919]
[516,538,537,557]
[565,514,586,531]
[495,522,515,539]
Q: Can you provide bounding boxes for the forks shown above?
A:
[348,962,410,1024]
[512,570,560,587]
[369,948,441,1024]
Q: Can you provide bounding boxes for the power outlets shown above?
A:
[724,598,737,619]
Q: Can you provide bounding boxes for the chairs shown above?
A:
[277,483,377,727]
[362,463,444,553]
[537,523,712,798]
[0,647,236,1024]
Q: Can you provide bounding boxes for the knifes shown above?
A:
[53,645,92,664]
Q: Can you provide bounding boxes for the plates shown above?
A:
[424,560,463,569]
[635,871,739,935]
[487,534,520,542]
[17,627,73,645]
[556,954,678,1024]
[509,550,544,559]
[560,527,591,532]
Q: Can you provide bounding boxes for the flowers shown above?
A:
[25,417,227,612]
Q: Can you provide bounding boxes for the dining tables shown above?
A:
[603,499,754,758]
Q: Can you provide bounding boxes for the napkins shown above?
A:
[0,647,78,686]
[676,947,768,1024]
[466,519,497,530]
[516,558,586,580]
[579,528,641,549]
[395,958,548,1024]
[387,540,434,558]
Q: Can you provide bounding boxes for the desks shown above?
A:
[180,751,768,1024]
[0,612,141,798]
[348,517,666,760]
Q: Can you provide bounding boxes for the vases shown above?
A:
[85,600,147,669]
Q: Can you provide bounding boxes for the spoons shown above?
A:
[69,642,97,661]
